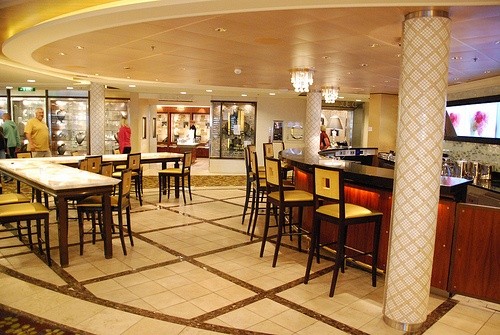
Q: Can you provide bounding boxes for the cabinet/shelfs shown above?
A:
[11,96,45,139]
[50,99,88,156]
[105,97,131,155]
[157,106,210,159]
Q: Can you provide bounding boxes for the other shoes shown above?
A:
[4,177,13,183]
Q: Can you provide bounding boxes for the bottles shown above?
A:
[58,145,65,155]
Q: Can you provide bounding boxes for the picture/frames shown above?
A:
[273,119,285,144]
[152,117,156,138]
[142,116,146,139]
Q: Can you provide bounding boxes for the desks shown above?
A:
[176,142,200,164]
[0,158,123,268]
[39,152,185,199]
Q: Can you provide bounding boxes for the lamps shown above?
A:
[320,85,340,104]
[288,66,315,94]
[326,117,344,147]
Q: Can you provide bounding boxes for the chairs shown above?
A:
[0,202,51,269]
[242,140,383,297]
[157,151,193,205]
[54,152,144,256]
[15,150,32,158]
[0,192,34,251]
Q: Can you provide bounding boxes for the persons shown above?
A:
[0,126,13,183]
[320,126,330,147]
[2,112,22,158]
[24,108,52,157]
[116,115,131,154]
